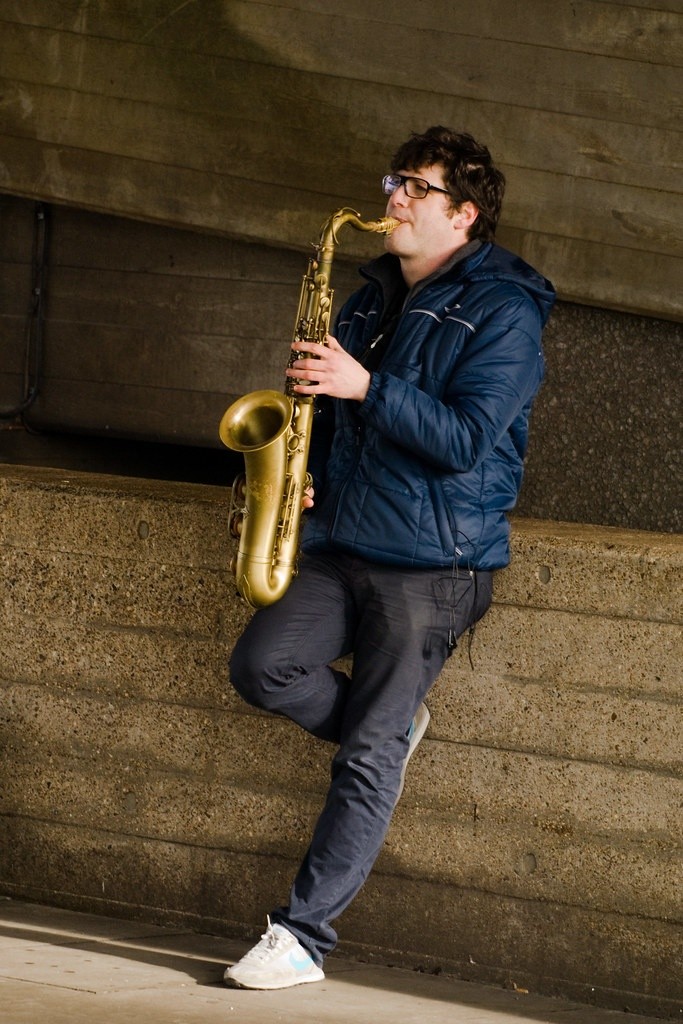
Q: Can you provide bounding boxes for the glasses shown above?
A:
[382,173,451,199]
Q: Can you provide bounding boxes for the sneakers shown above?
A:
[390,702,430,808]
[224,914,325,990]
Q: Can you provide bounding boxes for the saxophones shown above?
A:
[216,205,401,608]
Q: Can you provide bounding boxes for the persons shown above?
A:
[220,122,556,991]
